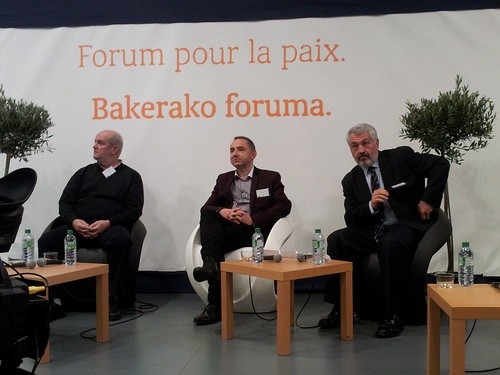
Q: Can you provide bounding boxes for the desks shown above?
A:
[6,260,108,364]
[220,254,353,357]
[427,283,500,375]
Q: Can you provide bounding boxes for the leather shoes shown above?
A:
[376,313,405,338]
[317,303,357,328]
[194,304,221,325]
[193,256,218,282]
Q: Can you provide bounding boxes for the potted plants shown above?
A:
[0,84,56,253]
[398,75,495,284]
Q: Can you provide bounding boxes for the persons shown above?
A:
[317,124,450,338]
[38,130,144,321]
[193,136,291,324]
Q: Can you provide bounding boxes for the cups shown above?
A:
[307,253,313,263]
[8,243,23,260]
[241,250,252,262]
[324,253,331,263]
[435,273,455,288]
[43,252,58,262]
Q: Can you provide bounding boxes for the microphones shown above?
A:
[263,254,282,263]
[297,252,313,262]
[36,258,63,267]
[10,259,36,269]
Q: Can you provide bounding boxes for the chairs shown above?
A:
[43,214,147,313]
[0,258,50,375]
[186,214,295,313]
[324,208,452,325]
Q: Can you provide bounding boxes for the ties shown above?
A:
[367,166,386,246]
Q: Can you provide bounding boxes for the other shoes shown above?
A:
[109,295,121,322]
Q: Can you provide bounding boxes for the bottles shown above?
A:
[273,250,281,263]
[296,250,306,262]
[313,228,326,264]
[458,242,474,286]
[22,229,35,260]
[64,230,76,266]
[252,228,264,262]
